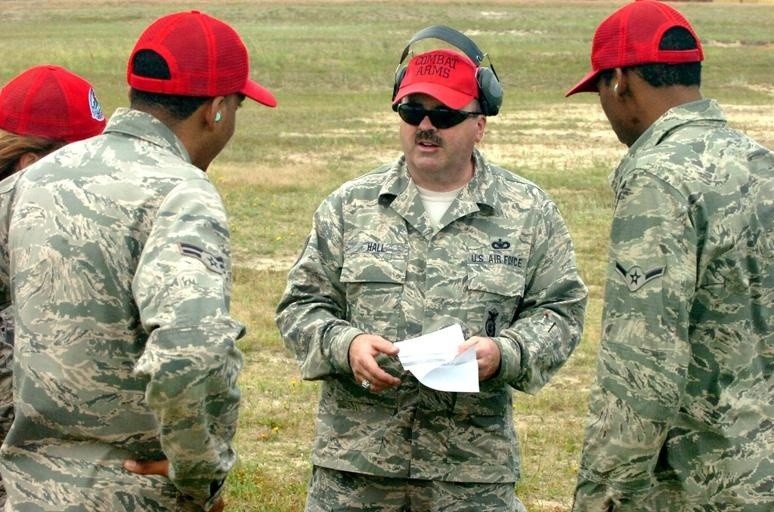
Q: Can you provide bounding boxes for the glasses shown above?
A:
[397,101,480,131]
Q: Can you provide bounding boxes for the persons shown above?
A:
[564,2,773,510]
[1,64,111,182]
[271,24,591,512]
[1,9,280,512]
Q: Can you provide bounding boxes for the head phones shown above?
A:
[392,26,503,116]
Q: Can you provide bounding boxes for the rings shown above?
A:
[361,377,371,390]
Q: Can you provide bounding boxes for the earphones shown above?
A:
[613,83,619,94]
[215,112,222,123]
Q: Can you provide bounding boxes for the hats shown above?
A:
[563,0,706,99]
[125,8,277,109]
[0,63,108,145]
[391,48,483,114]
